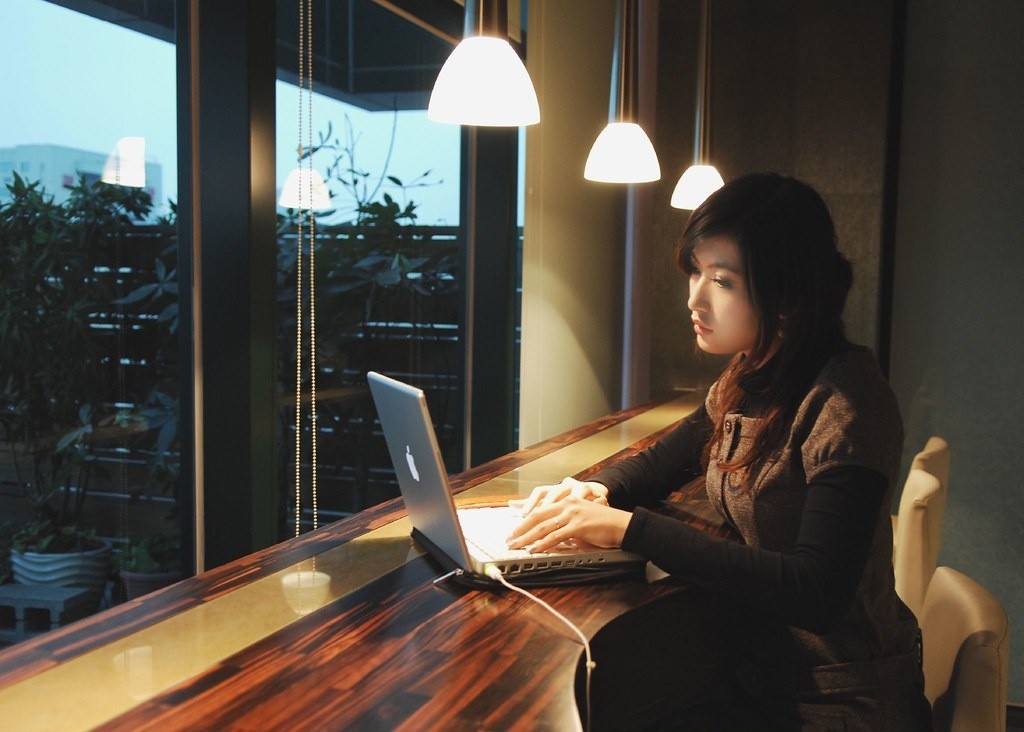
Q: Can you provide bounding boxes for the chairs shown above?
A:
[891,435,1009,732]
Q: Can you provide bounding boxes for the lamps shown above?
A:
[426,0,541,128]
[673,0,727,211]
[580,0,663,185]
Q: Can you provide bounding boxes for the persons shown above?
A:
[506,171,934,732]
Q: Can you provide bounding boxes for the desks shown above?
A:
[0,389,742,732]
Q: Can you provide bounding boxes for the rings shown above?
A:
[551,517,560,529]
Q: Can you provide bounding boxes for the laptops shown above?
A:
[365,371,643,576]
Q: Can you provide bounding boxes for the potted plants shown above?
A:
[0,158,182,618]
